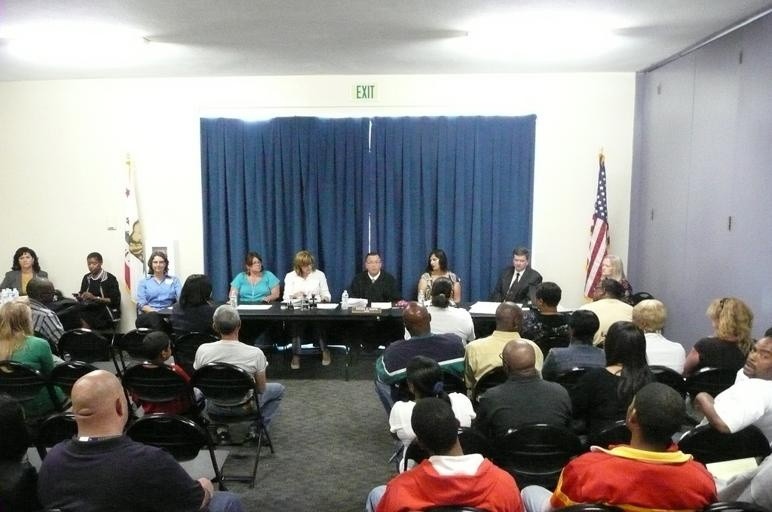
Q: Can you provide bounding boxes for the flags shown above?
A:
[583,152,612,300]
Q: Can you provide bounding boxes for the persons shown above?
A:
[463,301,544,399]
[37,369,247,511]
[520,382,718,512]
[170,274,221,341]
[574,278,634,347]
[388,355,477,474]
[683,296,757,403]
[678,327,772,466]
[403,277,477,348]
[374,301,466,416]
[0,394,37,512]
[193,304,285,445]
[542,310,606,382]
[72,252,121,328]
[364,397,525,511]
[521,282,573,361]
[632,299,687,376]
[1,296,68,432]
[348,252,409,351]
[569,321,658,431]
[122,330,205,416]
[712,447,771,511]
[282,250,332,369]
[592,254,633,307]
[225,252,280,351]
[472,339,571,491]
[135,251,182,335]
[26,276,98,370]
[417,249,462,304]
[491,247,542,302]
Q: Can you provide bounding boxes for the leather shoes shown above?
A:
[291,356,300,369]
[243,432,272,447]
[218,431,231,447]
[322,350,332,366]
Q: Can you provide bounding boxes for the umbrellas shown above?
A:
[119,155,146,336]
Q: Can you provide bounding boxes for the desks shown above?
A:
[156,303,572,381]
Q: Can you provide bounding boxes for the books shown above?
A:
[316,302,339,310]
[469,301,523,315]
[0,246,48,295]
[236,304,272,311]
[351,305,381,315]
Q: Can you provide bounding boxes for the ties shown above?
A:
[504,272,520,302]
[371,278,375,286]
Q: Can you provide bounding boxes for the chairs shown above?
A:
[631,291,655,308]
[554,365,595,393]
[544,506,622,512]
[683,366,737,403]
[492,422,583,494]
[677,424,771,465]
[136,303,139,319]
[649,365,686,402]
[123,413,227,493]
[418,503,481,512]
[34,413,78,461]
[187,362,275,487]
[390,369,468,402]
[57,328,128,399]
[116,328,176,373]
[174,333,222,374]
[0,360,61,413]
[32,330,57,356]
[471,366,540,411]
[404,428,492,472]
[588,420,633,451]
[47,361,129,419]
[121,364,206,449]
[694,502,763,512]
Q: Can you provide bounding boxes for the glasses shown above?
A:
[499,353,511,367]
[301,263,315,268]
[367,259,383,264]
[248,260,263,266]
[592,285,605,294]
[43,289,61,300]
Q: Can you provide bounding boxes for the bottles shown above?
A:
[417,289,425,306]
[230,290,237,309]
[0,288,19,302]
[341,290,349,310]
